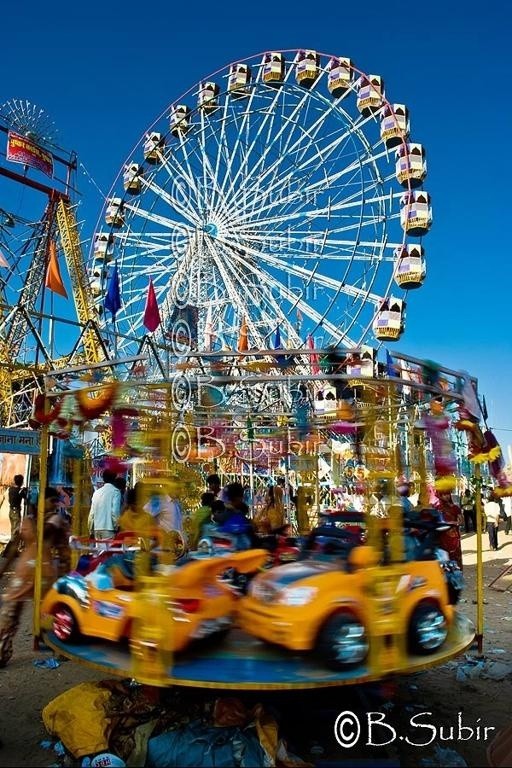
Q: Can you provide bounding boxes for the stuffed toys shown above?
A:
[38,526,271,665]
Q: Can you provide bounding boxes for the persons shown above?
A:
[0,468,420,556]
[499,491,511,535]
[483,495,501,551]
[431,478,464,572]
[1,485,67,667]
[460,489,477,534]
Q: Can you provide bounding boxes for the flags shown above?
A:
[45,239,70,299]
[387,350,397,377]
[101,266,122,314]
[274,324,281,350]
[306,333,320,374]
[236,315,250,361]
[143,279,162,333]
